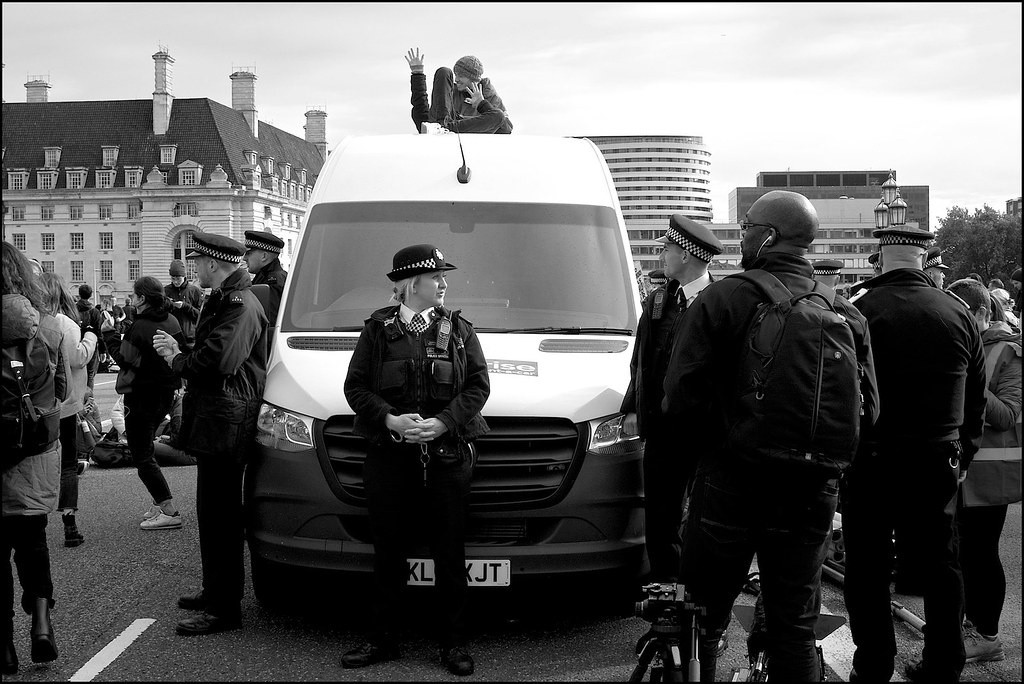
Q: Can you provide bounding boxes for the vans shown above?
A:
[249,131,647,605]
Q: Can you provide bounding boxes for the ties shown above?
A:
[676,286,686,309]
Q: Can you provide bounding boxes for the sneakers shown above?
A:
[139,503,183,530]
[961,619,1005,663]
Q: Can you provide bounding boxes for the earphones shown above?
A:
[763,235,773,245]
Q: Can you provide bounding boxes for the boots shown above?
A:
[62,511,85,547]
[29,597,58,663]
[0,620,19,674]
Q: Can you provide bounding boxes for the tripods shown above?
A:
[628,634,688,683]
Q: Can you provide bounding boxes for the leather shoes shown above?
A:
[178,590,207,610]
[716,629,729,658]
[341,639,402,668]
[439,637,475,677]
[175,611,244,637]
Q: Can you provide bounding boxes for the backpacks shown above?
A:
[0,310,62,452]
[723,268,863,462]
[86,425,135,468]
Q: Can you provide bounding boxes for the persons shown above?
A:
[404,47,513,134]
[619,190,1022,681]
[342,243,490,677]
[0,230,288,672]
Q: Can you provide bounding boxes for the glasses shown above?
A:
[739,218,780,240]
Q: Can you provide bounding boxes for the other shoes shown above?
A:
[904,659,959,683]
[77,461,88,475]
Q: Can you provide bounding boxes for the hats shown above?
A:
[869,225,949,271]
[648,269,669,285]
[387,243,457,281]
[655,213,724,264]
[185,233,247,264]
[168,260,186,277]
[242,230,284,255]
[1011,269,1022,283]
[813,260,845,277]
